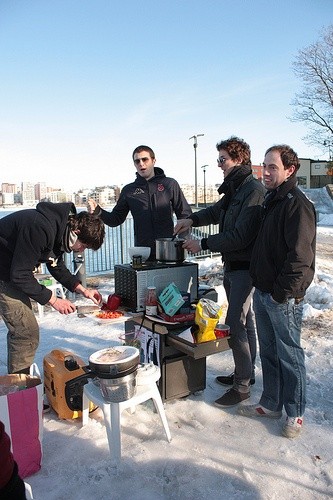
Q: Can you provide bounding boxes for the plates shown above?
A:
[144,314,179,324]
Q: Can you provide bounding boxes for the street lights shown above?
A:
[202,165,210,207]
[322,139,331,164]
[189,134,204,207]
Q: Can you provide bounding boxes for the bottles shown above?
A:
[145,286,157,315]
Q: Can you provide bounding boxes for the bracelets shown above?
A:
[52,298,57,306]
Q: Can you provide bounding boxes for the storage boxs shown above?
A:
[159,282,185,317]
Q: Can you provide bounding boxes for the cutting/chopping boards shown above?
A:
[74,304,133,324]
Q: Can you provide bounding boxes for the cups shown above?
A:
[131,255,142,269]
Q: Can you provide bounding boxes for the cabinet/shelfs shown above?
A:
[124,316,231,402]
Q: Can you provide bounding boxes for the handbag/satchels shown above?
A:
[0,363,43,478]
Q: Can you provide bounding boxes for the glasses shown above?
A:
[135,157,153,164]
[217,157,237,163]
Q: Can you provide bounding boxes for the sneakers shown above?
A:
[281,414,304,436]
[214,388,250,407]
[217,374,256,386]
[237,403,282,417]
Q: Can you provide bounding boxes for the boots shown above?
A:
[11,367,50,414]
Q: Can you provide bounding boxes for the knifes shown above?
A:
[60,306,99,313]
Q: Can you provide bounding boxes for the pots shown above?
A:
[88,363,139,403]
[156,237,187,261]
[88,346,139,375]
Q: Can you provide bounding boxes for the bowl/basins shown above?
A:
[214,321,230,340]
[128,247,151,263]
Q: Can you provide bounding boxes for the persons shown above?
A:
[0,202,106,413]
[236,144,317,437]
[88,146,193,261]
[174,136,267,408]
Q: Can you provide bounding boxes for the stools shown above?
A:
[37,277,66,317]
[82,381,171,463]
